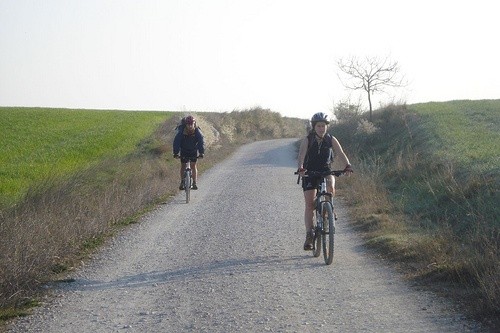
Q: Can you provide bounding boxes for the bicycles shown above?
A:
[306,126,312,135]
[174,155,202,202]
[294,168,355,266]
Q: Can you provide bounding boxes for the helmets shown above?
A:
[185,115,195,124]
[311,112,329,124]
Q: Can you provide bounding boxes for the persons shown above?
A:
[174,116,204,190]
[298,112,351,250]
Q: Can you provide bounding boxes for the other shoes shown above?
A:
[179,180,186,189]
[193,183,198,189]
[304,231,315,250]
